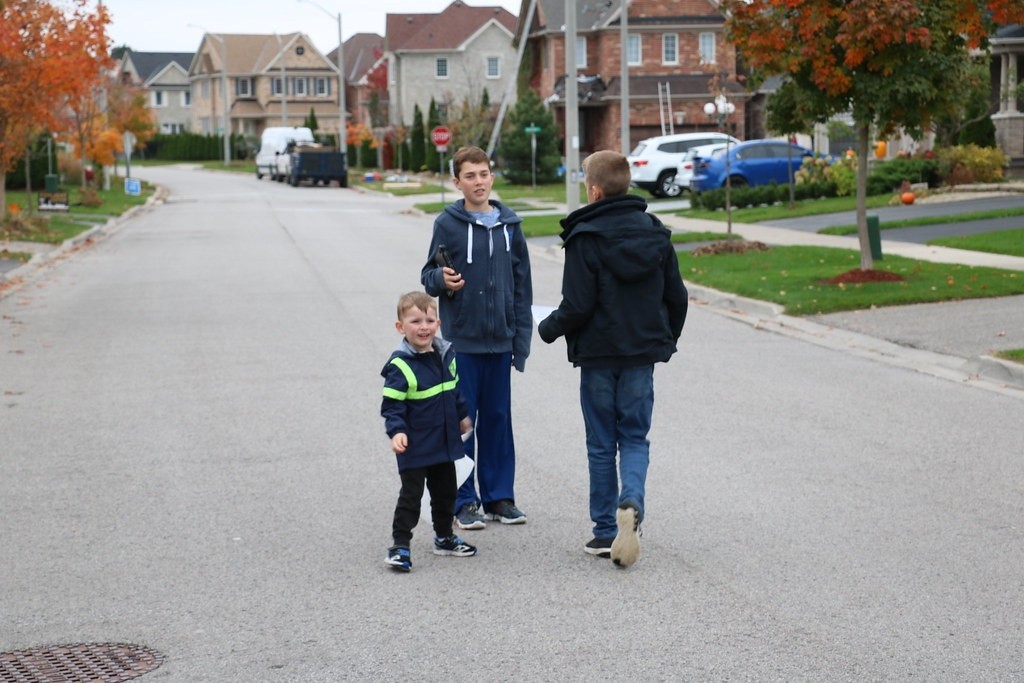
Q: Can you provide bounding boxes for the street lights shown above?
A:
[295,1,349,172]
[703,95,736,133]
[184,20,231,167]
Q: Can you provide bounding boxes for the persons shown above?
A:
[85,165,98,188]
[420,145,533,531]
[538,149,689,570]
[380,291,477,573]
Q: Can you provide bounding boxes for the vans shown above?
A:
[254,125,318,182]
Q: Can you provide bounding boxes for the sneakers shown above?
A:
[384,546,413,572]
[483,499,527,524]
[584,538,616,559]
[432,535,477,557]
[610,503,642,568]
[454,501,486,530]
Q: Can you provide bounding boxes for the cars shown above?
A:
[675,140,741,196]
[277,138,349,188]
[687,137,843,193]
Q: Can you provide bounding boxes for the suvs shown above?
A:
[625,131,740,198]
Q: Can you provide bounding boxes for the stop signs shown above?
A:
[430,126,453,147]
